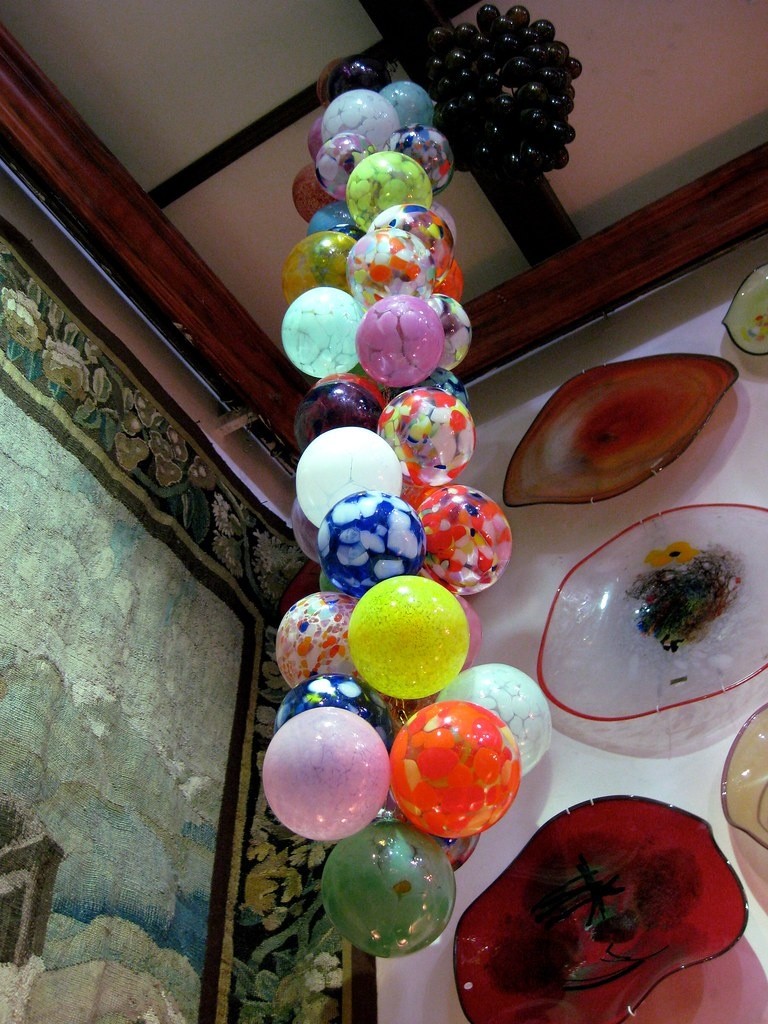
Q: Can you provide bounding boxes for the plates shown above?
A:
[720,706,768,852]
[720,263,767,358]
[451,794,750,1024]
[502,353,740,508]
[537,504,767,721]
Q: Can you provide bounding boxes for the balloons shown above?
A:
[262,4,584,959]
